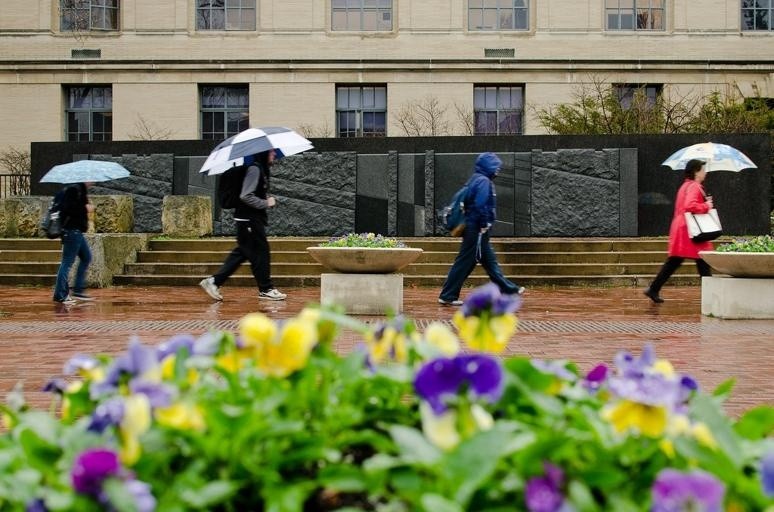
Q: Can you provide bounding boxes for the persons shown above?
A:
[40,179,98,305]
[438,150,527,307]
[642,158,721,304]
[197,145,290,303]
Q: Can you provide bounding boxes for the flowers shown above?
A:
[311,231,409,251]
[696,232,773,254]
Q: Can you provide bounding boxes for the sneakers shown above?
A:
[438,298,463,305]
[199,277,223,300]
[258,289,287,300]
[643,287,664,303]
[55,293,76,305]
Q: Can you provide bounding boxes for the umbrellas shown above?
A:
[197,124,313,177]
[39,158,132,187]
[660,139,758,176]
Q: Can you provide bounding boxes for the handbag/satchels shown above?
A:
[684,209,723,243]
[441,186,469,237]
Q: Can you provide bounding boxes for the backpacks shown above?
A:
[218,162,248,209]
[42,186,82,238]
